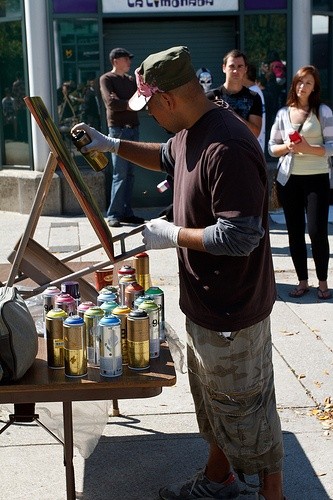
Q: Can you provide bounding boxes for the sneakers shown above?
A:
[158,464,239,500]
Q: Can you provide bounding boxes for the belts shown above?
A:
[121,123,140,129]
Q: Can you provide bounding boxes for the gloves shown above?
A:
[142,218,184,249]
[70,122,120,155]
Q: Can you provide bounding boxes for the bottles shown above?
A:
[288,128,302,144]
[70,129,110,172]
[43,251,166,382]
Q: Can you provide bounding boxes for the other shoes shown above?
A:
[121,215,144,224]
[108,220,120,227]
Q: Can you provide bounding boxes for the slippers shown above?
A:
[289,286,308,297]
[316,287,328,300]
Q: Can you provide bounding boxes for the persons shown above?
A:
[206,48,263,138]
[2,72,29,145]
[257,60,287,132]
[197,65,210,92]
[97,47,145,228]
[69,45,290,500]
[69,77,100,131]
[243,62,267,154]
[268,65,333,301]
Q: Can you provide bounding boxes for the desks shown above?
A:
[0,262,188,500]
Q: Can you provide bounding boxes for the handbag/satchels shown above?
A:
[0,280,40,383]
[271,179,283,209]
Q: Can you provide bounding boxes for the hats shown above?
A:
[109,48,135,59]
[128,46,195,111]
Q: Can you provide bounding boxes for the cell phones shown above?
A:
[289,131,302,144]
